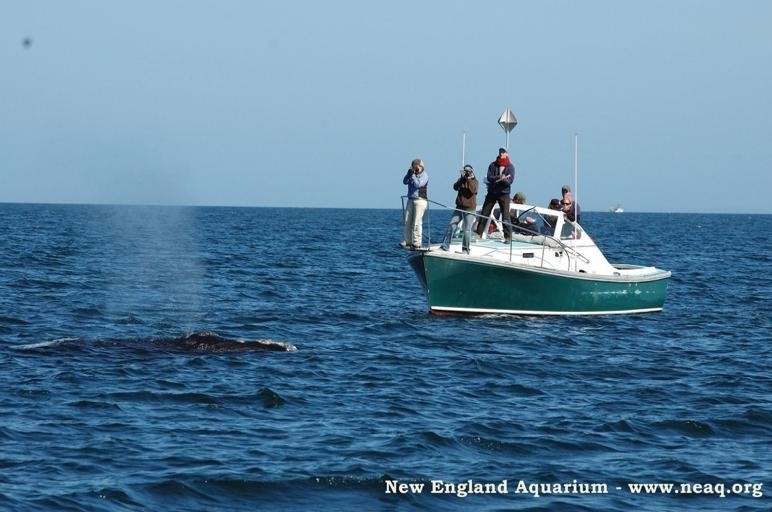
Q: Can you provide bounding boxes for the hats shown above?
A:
[562,185,570,192]
[499,147,508,153]
[551,199,561,205]
[515,192,525,204]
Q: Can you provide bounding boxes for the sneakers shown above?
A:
[412,243,422,248]
[399,241,410,246]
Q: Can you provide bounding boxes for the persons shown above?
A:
[399,159,428,249]
[509,192,538,235]
[439,165,479,254]
[544,186,581,235]
[476,146,515,243]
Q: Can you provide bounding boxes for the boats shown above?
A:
[608,207,624,214]
[400,108,672,320]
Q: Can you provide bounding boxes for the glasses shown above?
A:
[563,202,570,205]
